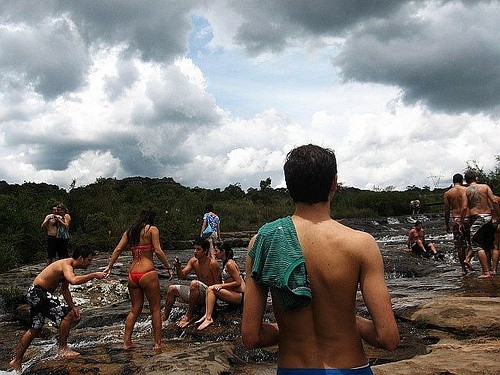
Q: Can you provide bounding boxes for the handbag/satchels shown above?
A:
[202,225,213,240]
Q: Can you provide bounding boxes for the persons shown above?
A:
[102,207,174,351]
[56,204,71,260]
[407,221,439,257]
[201,204,221,247]
[242,146,399,374]
[9,244,106,372]
[465,170,496,278]
[443,173,475,274]
[41,206,60,266]
[161,239,221,327]
[196,242,251,330]
[490,194,500,275]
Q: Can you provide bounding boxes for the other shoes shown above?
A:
[425,250,430,258]
[434,253,445,259]
